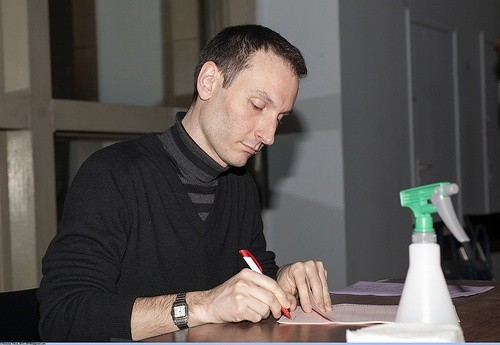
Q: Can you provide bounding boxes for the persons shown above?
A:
[35,25,333,342]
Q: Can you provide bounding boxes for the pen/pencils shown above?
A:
[239,250,292,320]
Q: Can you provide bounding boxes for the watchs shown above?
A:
[170,292,190,330]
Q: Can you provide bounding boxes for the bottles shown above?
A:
[396,181,471,330]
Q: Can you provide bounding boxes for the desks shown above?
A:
[141,277,500,345]
